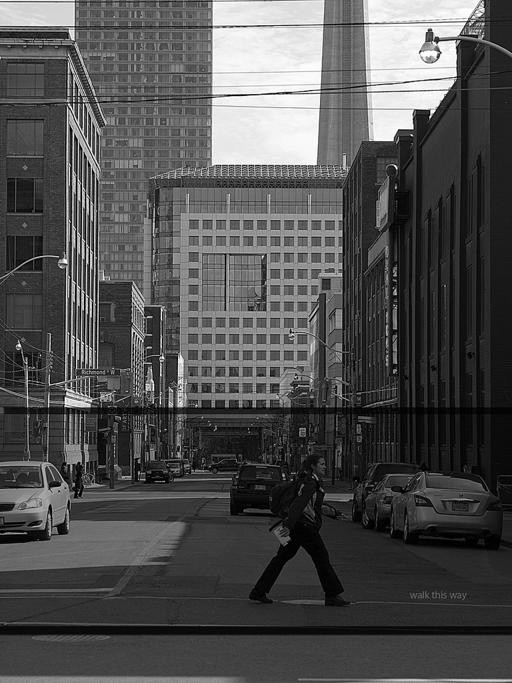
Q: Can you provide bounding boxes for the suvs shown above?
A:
[230,463,284,515]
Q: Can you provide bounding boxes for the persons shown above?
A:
[134,456,147,482]
[59,462,84,500]
[249,453,352,607]
[6,469,16,480]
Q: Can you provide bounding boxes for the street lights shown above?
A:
[127,354,164,481]
[418,27,511,63]
[15,340,30,458]
[286,329,356,475]
[179,404,217,461]
[1,254,70,284]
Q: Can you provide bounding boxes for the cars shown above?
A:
[145,458,192,485]
[353,461,502,549]
[1,461,70,539]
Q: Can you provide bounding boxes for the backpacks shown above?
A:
[269,478,320,531]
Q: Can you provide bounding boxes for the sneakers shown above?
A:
[324,592,350,606]
[248,588,273,603]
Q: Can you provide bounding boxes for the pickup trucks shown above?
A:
[207,458,251,474]
